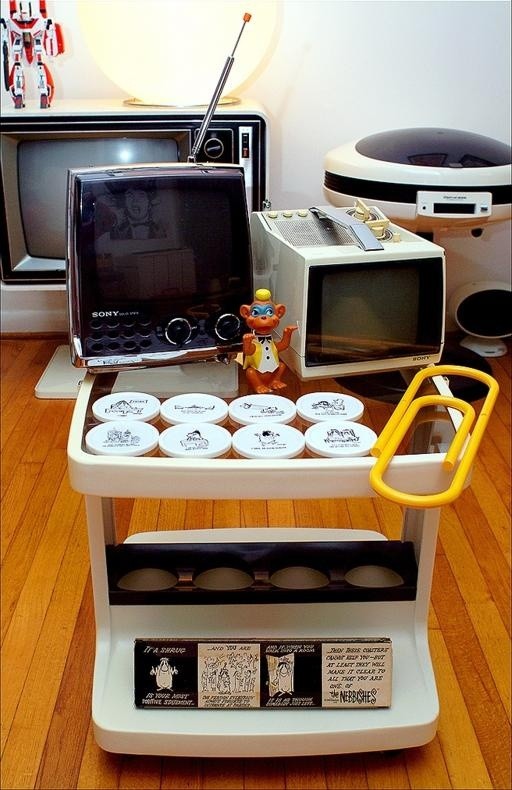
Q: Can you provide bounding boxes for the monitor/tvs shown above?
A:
[67,161,251,374]
[0,108,270,336]
